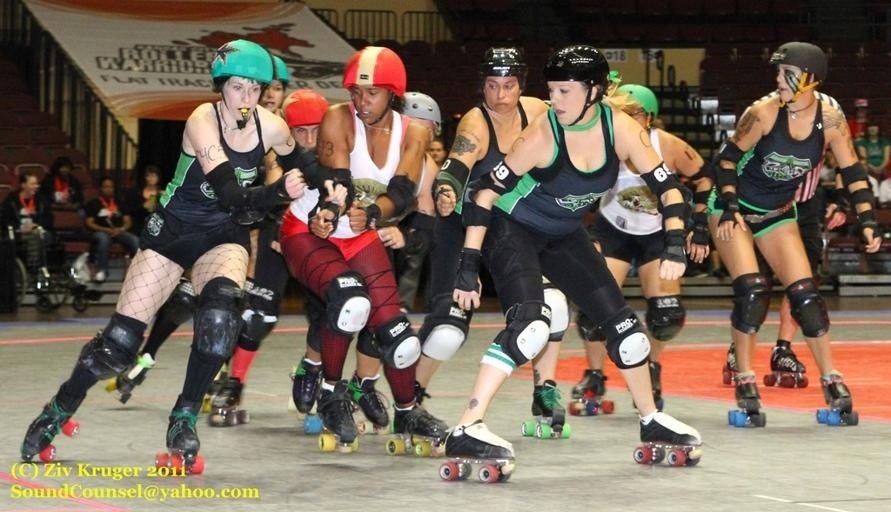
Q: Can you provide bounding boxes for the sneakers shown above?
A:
[96,270,109,283]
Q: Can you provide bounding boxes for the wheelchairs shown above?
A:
[2,224,88,313]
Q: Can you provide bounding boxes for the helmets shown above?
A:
[544,46,611,86]
[619,83,657,117]
[270,56,291,81]
[771,42,830,79]
[400,92,442,128]
[212,40,274,85]
[482,44,528,77]
[283,91,328,128]
[342,46,406,97]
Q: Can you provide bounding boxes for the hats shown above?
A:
[855,98,870,110]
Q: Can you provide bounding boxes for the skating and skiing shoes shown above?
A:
[816,374,859,426]
[413,382,428,407]
[648,359,665,410]
[211,375,250,425]
[524,381,571,436]
[384,403,451,456]
[340,372,390,435]
[106,354,152,403]
[22,384,84,463]
[570,370,616,416]
[764,343,810,388]
[287,358,324,415]
[202,368,229,411]
[721,343,739,383]
[729,374,767,429]
[155,396,206,474]
[635,412,704,466]
[304,377,361,453]
[441,419,518,483]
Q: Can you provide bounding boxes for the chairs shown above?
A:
[821,213,866,274]
[667,0,705,22]
[0,61,17,76]
[456,55,481,67]
[459,80,484,99]
[746,19,777,44]
[65,148,87,164]
[645,22,681,44]
[524,82,551,100]
[0,72,27,93]
[26,125,59,145]
[526,56,549,82]
[53,210,91,254]
[404,63,425,81]
[428,55,452,70]
[869,98,891,113]
[15,163,48,184]
[0,126,27,145]
[0,165,12,184]
[836,98,856,118]
[680,22,711,44]
[438,96,467,118]
[432,41,463,64]
[347,38,371,51]
[0,184,12,204]
[373,38,404,57]
[15,91,36,111]
[772,0,809,20]
[522,40,551,56]
[867,209,891,273]
[604,0,635,22]
[611,23,646,45]
[80,187,98,208]
[806,0,891,100]
[737,0,771,20]
[0,110,22,126]
[424,80,461,99]
[776,19,807,43]
[869,113,891,135]
[72,163,95,189]
[432,0,605,40]
[0,144,36,165]
[713,21,746,43]
[705,0,736,21]
[401,57,425,73]
[635,0,668,22]
[478,36,507,55]
[404,39,431,62]
[426,64,450,82]
[0,90,13,111]
[21,110,59,125]
[698,43,777,145]
[35,144,63,164]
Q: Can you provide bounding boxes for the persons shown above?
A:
[427,140,448,165]
[710,238,726,277]
[853,118,891,181]
[568,83,712,417]
[819,149,838,184]
[879,178,890,208]
[131,165,165,236]
[847,99,874,139]
[438,45,704,482]
[206,88,358,425]
[41,157,83,210]
[706,42,884,427]
[20,39,348,477]
[722,181,848,389]
[0,174,67,279]
[104,54,294,413]
[413,47,572,439]
[84,176,140,282]
[289,92,441,433]
[279,46,451,457]
[860,157,880,208]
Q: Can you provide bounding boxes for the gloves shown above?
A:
[720,200,741,228]
[432,178,454,208]
[863,216,882,242]
[319,171,347,198]
[360,202,383,231]
[309,203,340,235]
[455,249,484,294]
[263,174,295,211]
[827,185,851,219]
[661,235,689,266]
[688,212,711,246]
[391,223,416,248]
